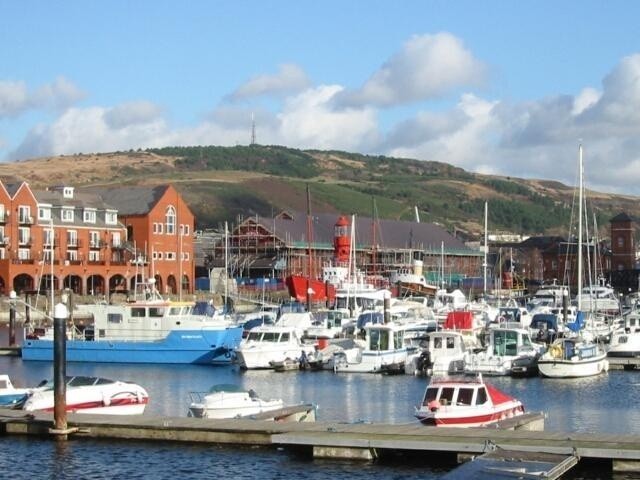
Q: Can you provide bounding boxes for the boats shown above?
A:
[0,373,39,406]
[20,372,149,415]
[185,388,284,420]
[411,370,526,427]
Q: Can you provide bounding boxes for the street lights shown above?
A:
[8,290,17,345]
